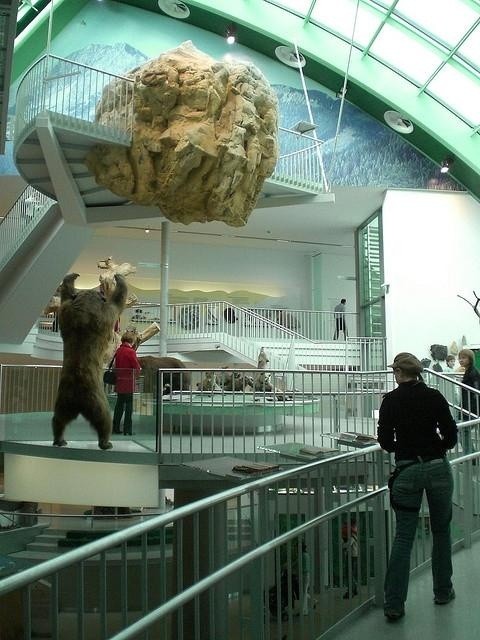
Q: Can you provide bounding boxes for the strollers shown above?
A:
[259,565,306,620]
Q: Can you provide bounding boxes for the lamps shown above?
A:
[224,22,237,45]
[333,79,350,100]
[275,44,307,69]
[438,155,456,175]
[157,0,192,19]
[382,108,414,136]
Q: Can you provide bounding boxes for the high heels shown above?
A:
[112,429,123,435]
[123,429,135,435]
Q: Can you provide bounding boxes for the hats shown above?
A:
[387,352,424,375]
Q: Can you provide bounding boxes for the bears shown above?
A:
[48,272,129,451]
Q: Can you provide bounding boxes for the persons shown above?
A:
[455,347,479,467]
[107,331,141,437]
[378,352,461,625]
[443,354,456,373]
[339,511,359,602]
[332,299,352,344]
[283,539,318,618]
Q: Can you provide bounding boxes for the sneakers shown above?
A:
[433,587,456,605]
[383,603,406,620]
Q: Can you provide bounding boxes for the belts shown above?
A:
[394,454,444,465]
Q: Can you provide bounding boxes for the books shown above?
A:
[232,460,280,477]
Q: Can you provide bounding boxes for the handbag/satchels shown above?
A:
[103,353,117,385]
